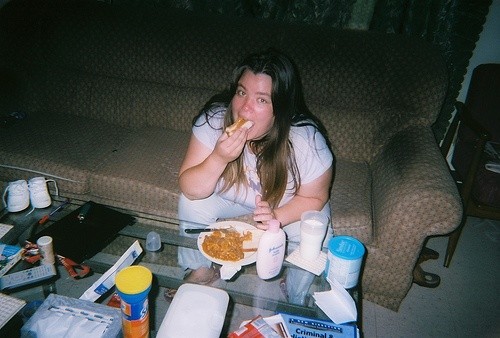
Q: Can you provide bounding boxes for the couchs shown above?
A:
[0,13,464,314]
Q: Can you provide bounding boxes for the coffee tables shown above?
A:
[0,191,364,338]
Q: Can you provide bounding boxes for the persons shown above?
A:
[164,49,334,304]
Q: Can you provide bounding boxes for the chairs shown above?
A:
[443,63,500,269]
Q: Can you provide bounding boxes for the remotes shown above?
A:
[1,263,58,291]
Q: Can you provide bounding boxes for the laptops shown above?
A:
[29,200,135,263]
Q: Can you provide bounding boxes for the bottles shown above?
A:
[324,236,365,289]
[255,219,287,280]
[37,235,56,266]
[115,266,152,338]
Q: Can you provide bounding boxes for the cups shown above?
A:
[300,210,329,258]
[146,232,161,251]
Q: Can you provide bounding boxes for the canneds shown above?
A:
[326,236,365,288]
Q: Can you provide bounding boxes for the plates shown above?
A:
[197,220,260,265]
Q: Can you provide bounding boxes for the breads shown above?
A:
[226,118,254,137]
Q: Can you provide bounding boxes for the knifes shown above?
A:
[185,228,227,233]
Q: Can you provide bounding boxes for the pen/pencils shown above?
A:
[38,199,71,224]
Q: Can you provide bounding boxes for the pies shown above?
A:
[202,230,252,261]
